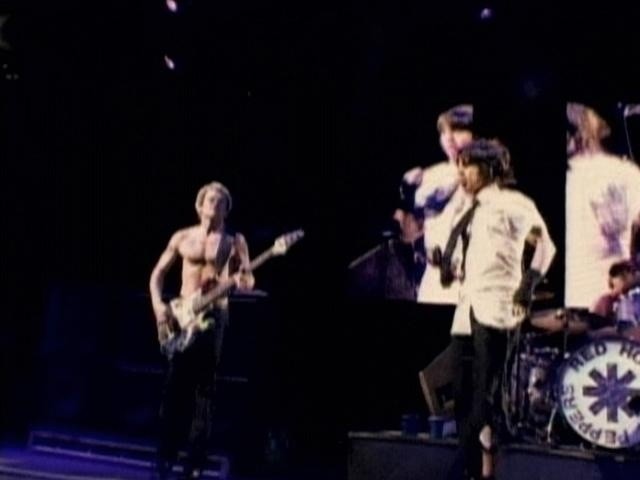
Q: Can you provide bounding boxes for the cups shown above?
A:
[428,416,446,439]
[403,415,418,436]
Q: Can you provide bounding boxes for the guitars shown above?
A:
[157,233,304,357]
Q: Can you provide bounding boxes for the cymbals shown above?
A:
[533,308,609,330]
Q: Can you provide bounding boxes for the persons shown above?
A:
[399,102,476,442]
[149,180,255,478]
[599,260,640,340]
[564,103,640,313]
[395,138,558,479]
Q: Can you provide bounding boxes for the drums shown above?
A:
[558,335,639,451]
[507,349,563,446]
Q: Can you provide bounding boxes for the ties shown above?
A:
[440,201,479,286]
